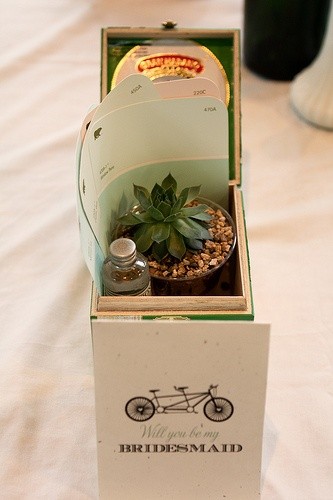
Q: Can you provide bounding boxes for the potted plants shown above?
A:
[114,171,236,297]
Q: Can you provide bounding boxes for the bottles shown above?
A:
[100,237,153,298]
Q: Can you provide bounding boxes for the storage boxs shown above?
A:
[89,26,254,321]
